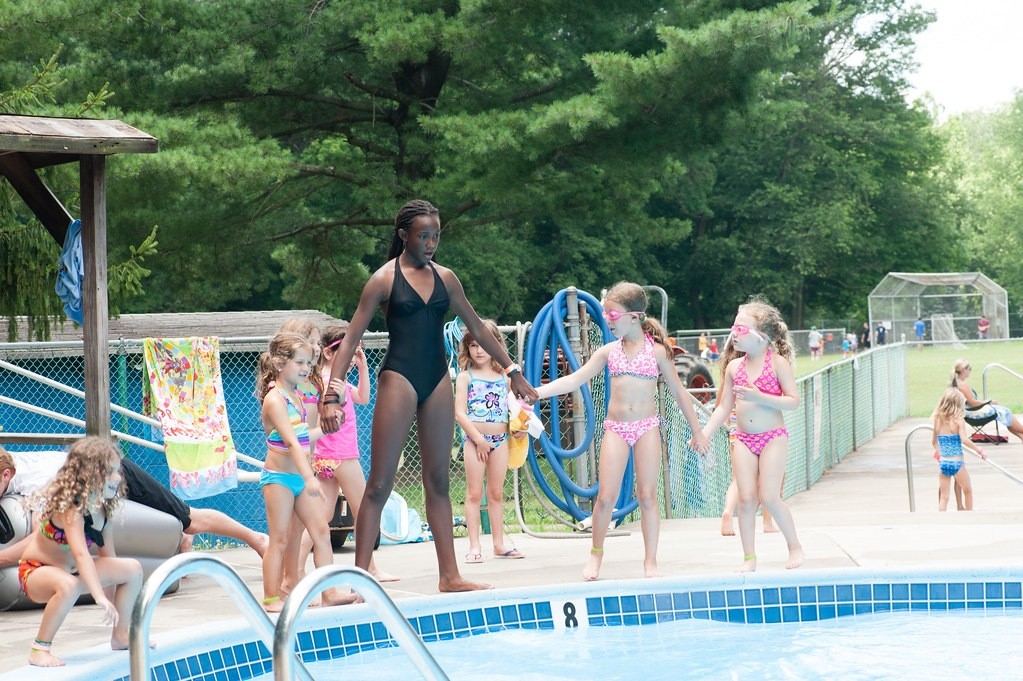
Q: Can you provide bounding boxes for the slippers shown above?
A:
[494,548,526,558]
[464,553,482,563]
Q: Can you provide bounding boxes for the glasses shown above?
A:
[964,367,972,371]
[731,325,764,341]
[601,311,645,321]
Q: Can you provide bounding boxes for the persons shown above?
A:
[524,280,712,581]
[699,332,720,363]
[256,329,357,612]
[687,293,805,573]
[273,319,400,606]
[947,357,1023,443]
[932,388,988,512]
[978,315,990,339]
[875,322,888,348]
[0,446,269,578]
[860,322,871,351]
[18,434,157,666]
[454,319,525,564]
[841,332,858,359]
[913,316,926,351]
[320,201,540,603]
[808,325,824,361]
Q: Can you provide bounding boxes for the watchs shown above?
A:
[507,365,521,377]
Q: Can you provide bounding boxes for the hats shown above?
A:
[811,325,816,330]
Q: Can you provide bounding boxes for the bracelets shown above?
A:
[324,400,340,405]
[977,447,984,454]
[504,363,516,374]
[325,393,339,398]
